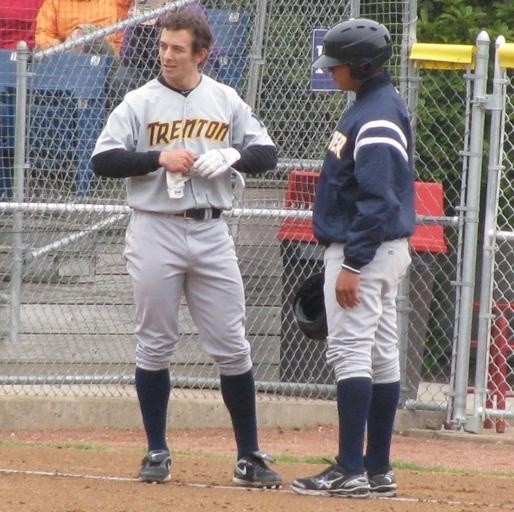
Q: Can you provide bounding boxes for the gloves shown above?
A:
[165,169,192,200]
[192,147,242,180]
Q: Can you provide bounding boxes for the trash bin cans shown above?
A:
[275,171,447,405]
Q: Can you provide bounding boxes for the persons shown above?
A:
[32,1,135,82]
[87,5,288,489]
[279,15,418,501]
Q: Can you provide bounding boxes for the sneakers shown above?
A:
[137,448,172,484]
[369,470,398,498]
[231,450,285,489]
[290,462,371,500]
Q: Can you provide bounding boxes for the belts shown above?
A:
[173,207,225,220]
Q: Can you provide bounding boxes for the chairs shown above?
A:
[203,8,250,85]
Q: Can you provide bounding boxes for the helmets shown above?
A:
[311,17,395,82]
[294,273,326,342]
